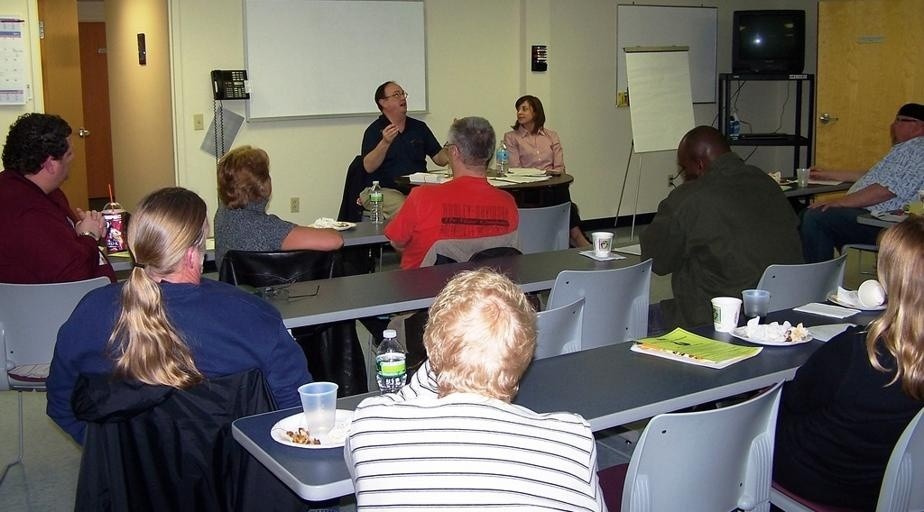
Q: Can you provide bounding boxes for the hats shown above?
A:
[898,103,924,121]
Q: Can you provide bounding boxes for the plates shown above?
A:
[308,222,356,230]
[729,323,813,346]
[778,180,793,185]
[826,289,890,311]
[271,408,355,450]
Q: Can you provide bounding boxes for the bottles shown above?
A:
[368,181,386,225]
[101,202,127,253]
[374,329,407,395]
[495,141,509,177]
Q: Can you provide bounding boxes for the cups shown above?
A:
[742,289,770,322]
[298,382,339,436]
[709,297,742,334]
[591,231,613,258]
[797,168,810,188]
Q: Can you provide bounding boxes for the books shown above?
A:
[409,170,443,184]
[629,325,765,370]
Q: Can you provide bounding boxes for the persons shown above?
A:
[343,265,608,512]
[0,113,117,284]
[44,186,315,512]
[639,125,805,337]
[383,116,520,270]
[801,103,924,262]
[770,213,923,512]
[502,95,592,248]
[211,143,344,275]
[361,81,458,219]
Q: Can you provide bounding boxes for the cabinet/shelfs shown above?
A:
[718,73,815,177]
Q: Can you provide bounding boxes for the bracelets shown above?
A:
[79,231,96,236]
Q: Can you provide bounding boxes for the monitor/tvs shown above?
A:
[731,9,806,79]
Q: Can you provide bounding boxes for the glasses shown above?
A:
[380,90,408,100]
[259,279,320,300]
[443,141,456,150]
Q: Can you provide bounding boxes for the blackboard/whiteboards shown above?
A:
[242,1,429,123]
[616,4,718,105]
